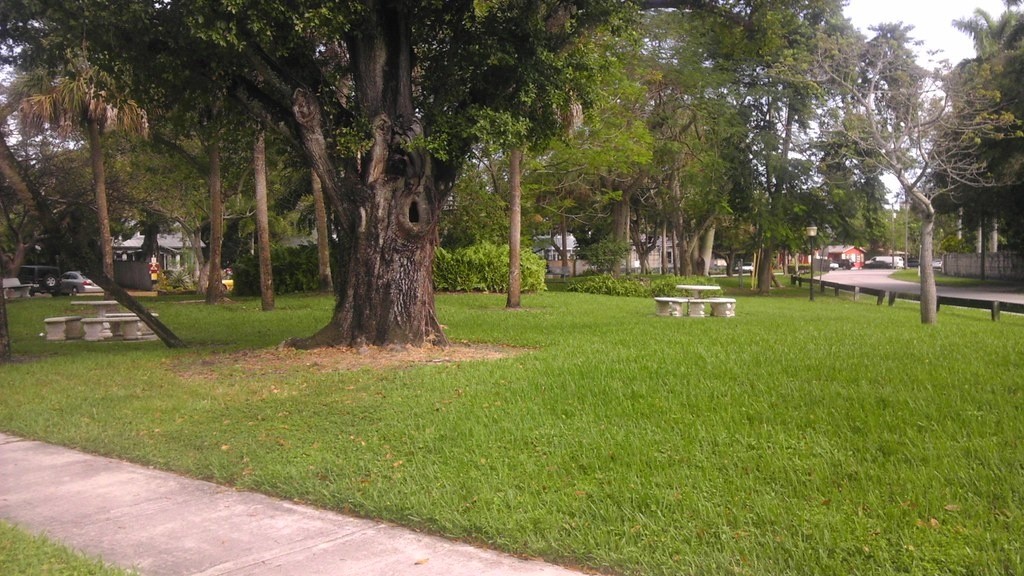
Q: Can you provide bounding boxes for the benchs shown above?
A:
[44,312,159,342]
[654,295,736,317]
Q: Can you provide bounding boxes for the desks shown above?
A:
[72,300,118,318]
[676,284,720,299]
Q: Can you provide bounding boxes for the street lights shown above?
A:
[806,224,817,302]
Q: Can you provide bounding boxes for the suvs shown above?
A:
[17,266,62,297]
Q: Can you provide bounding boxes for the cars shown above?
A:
[222,275,234,292]
[60,271,104,296]
